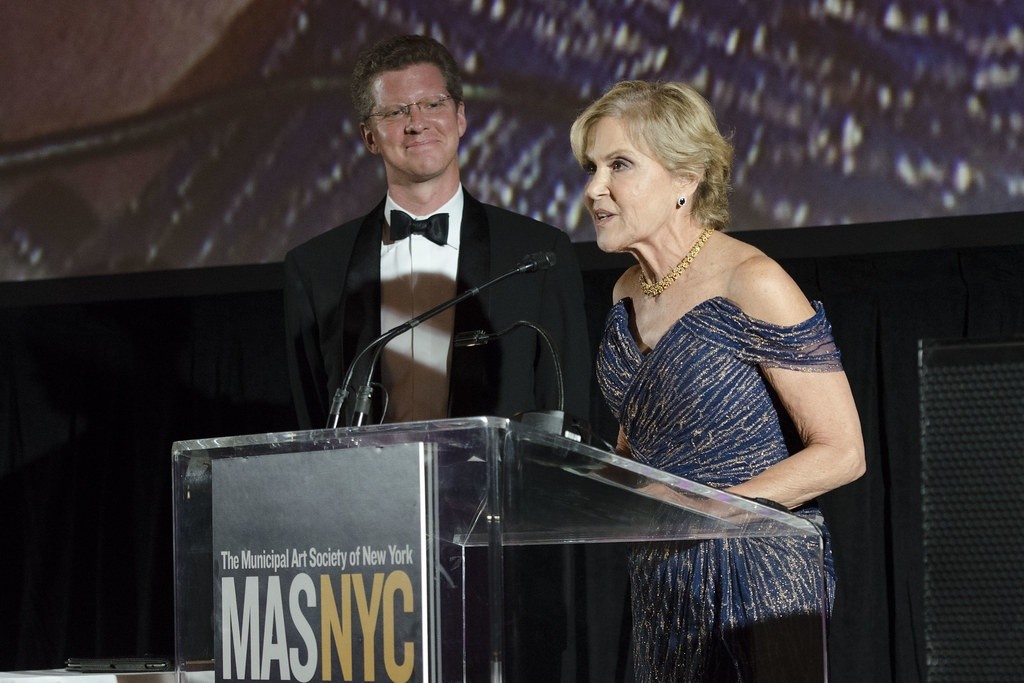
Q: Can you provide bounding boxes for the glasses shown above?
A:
[363,95,460,130]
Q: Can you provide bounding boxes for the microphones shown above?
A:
[325,246,565,430]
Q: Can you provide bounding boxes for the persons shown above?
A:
[278,32,594,683]
[568,81,868,683]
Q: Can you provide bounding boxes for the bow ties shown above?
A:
[389,209,449,245]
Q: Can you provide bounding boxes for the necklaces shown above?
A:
[639,223,718,298]
